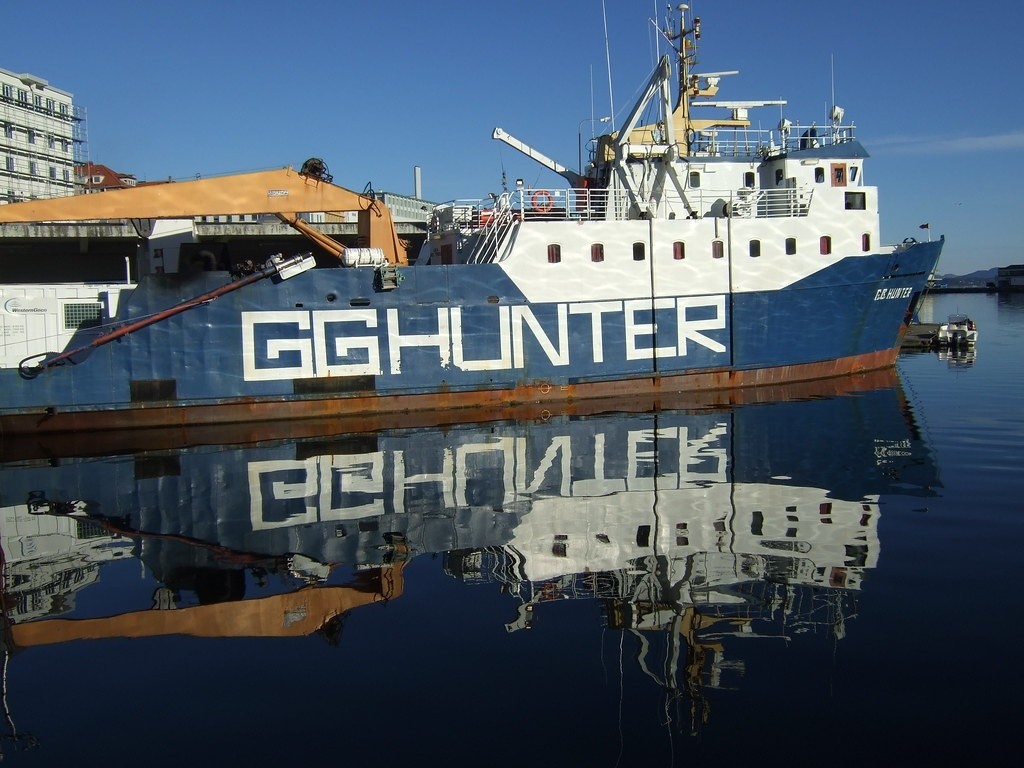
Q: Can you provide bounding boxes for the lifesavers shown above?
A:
[531,191,554,213]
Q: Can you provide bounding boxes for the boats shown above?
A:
[0,0,945,436]
[0,367,943,766]
[900,318,979,348]
